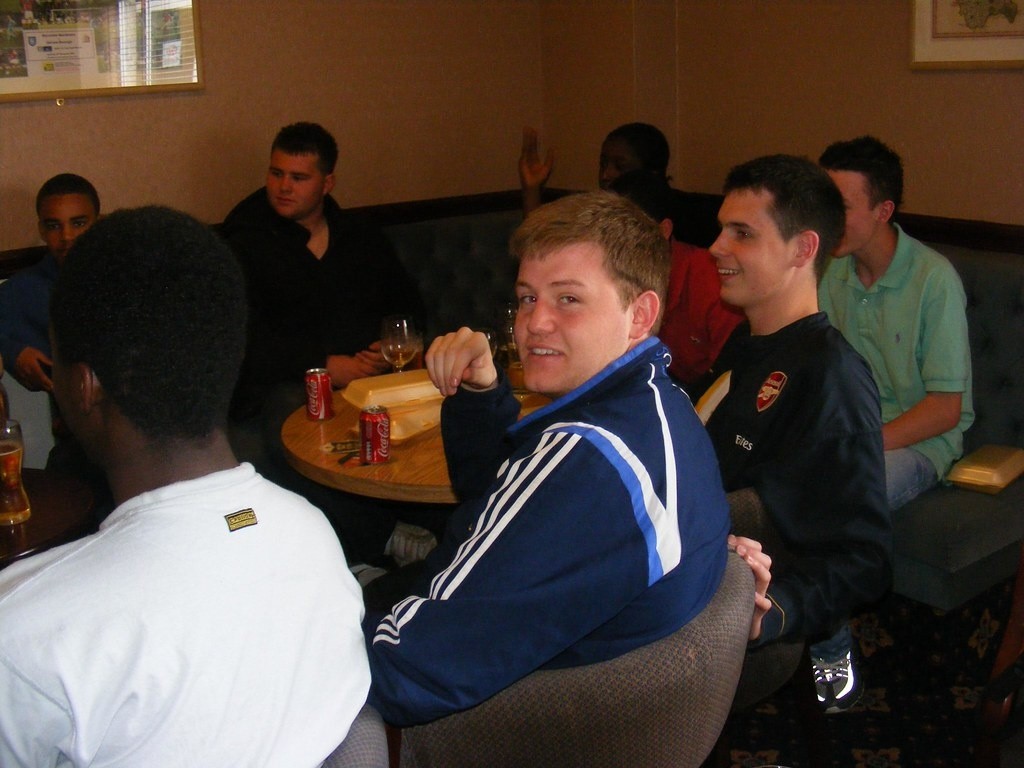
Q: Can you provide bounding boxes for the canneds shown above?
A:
[360,404,391,464]
[304,368,335,421]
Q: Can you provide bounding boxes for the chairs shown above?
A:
[321,701,390,768]
[398,544,756,768]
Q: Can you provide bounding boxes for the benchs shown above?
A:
[0,180,1024,767]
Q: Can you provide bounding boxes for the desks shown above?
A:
[279,367,559,506]
[0,468,97,572]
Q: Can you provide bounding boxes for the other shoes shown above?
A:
[350,564,387,587]
[387,519,439,565]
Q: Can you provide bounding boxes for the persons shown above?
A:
[513,121,745,382]
[811,136,976,713]
[0,173,100,391]
[364,193,729,725]
[695,155,890,645]
[0,206,373,768]
[220,123,426,387]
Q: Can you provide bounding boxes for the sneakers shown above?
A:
[810,648,865,714]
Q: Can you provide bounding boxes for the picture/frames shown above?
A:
[1,0,205,103]
[908,0,1024,71]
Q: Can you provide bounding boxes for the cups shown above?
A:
[379,314,419,373]
[1,420,30,525]
[469,326,497,360]
[506,323,525,387]
